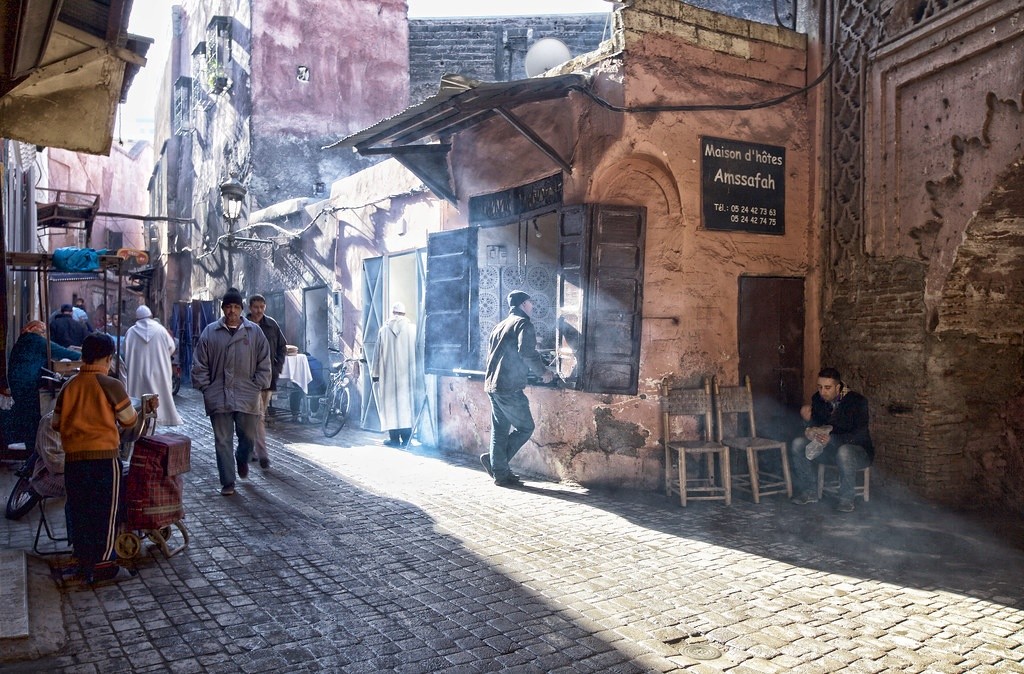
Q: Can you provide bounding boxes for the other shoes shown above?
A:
[221,482,235,494]
[260,458,267,468]
[311,411,318,418]
[289,415,302,422]
[237,461,249,478]
[252,458,258,461]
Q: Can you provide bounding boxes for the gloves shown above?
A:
[373,377,379,382]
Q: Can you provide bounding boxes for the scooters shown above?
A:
[95,327,181,396]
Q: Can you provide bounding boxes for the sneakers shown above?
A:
[838,498,855,512]
[480,454,494,478]
[494,469,520,485]
[792,489,818,504]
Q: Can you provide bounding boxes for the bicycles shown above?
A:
[318,348,368,437]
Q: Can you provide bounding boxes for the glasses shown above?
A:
[40,331,45,335]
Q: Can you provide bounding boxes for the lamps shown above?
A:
[220,171,302,255]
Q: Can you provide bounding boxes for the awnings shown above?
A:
[321,72,585,150]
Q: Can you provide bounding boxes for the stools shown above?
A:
[817,463,870,502]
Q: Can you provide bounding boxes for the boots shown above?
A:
[384,429,413,447]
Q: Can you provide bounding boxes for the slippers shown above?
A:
[96,565,139,584]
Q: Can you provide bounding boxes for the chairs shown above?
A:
[712,374,793,503]
[663,377,732,507]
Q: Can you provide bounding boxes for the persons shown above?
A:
[791,367,874,512]
[8,320,82,459]
[371,302,424,445]
[52,331,140,585]
[480,290,555,485]
[245,295,288,474]
[191,287,272,495]
[48,298,184,427]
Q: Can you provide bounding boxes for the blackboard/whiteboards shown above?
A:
[699,136,786,235]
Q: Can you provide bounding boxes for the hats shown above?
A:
[509,292,530,306]
[222,288,242,304]
[61,304,72,311]
[136,305,152,319]
[393,303,405,313]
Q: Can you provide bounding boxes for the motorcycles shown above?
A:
[5,366,152,521]
